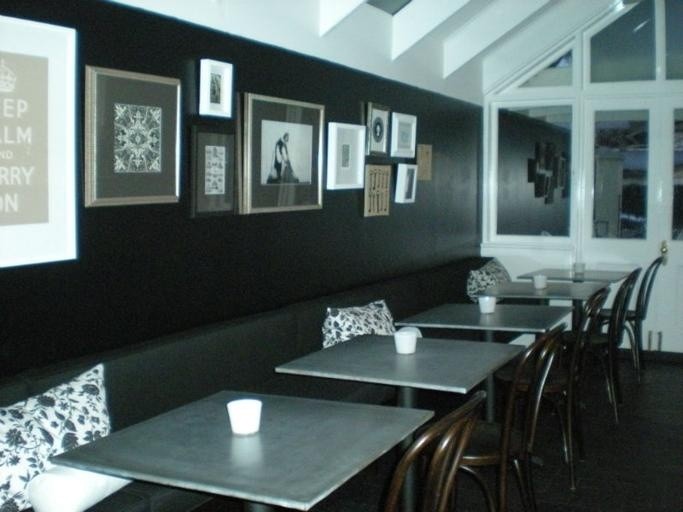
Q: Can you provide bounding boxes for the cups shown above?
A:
[573,274,585,283]
[531,274,547,290]
[478,313,498,330]
[224,397,264,436]
[227,435,264,470]
[535,287,548,297]
[574,263,585,274]
[477,295,495,314]
[393,330,416,355]
[395,356,415,379]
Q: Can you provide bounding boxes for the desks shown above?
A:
[481,278,605,394]
[277,334,524,507]
[52,390,426,509]
[397,300,574,446]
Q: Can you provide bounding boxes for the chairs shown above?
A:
[564,268,641,426]
[515,292,607,456]
[594,256,665,372]
[383,388,489,512]
[448,324,567,508]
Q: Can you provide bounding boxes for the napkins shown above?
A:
[467,259,512,302]
[322,301,397,347]
[1,363,132,510]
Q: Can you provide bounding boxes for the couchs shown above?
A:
[2,259,493,509]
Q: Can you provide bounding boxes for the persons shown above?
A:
[266,133,299,183]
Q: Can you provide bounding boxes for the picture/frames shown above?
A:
[0,9,87,276]
[187,56,236,120]
[327,123,365,190]
[242,93,325,215]
[84,64,182,207]
[368,102,391,158]
[391,112,417,158]
[189,123,237,219]
[393,163,417,204]
